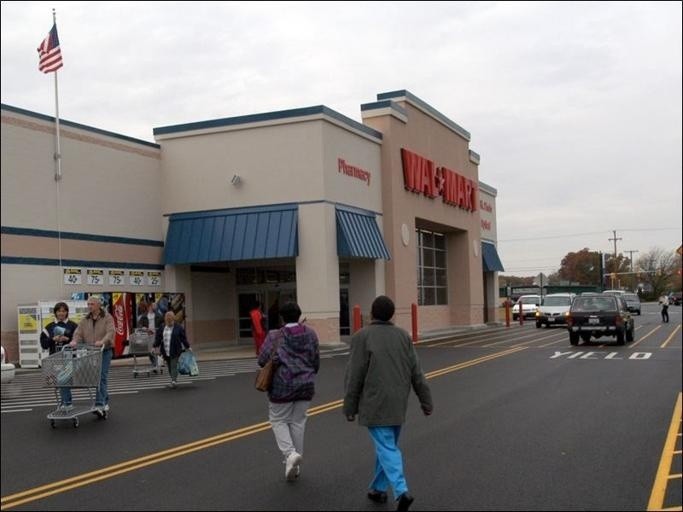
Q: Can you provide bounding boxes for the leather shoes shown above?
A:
[397,493,413,512]
[367,491,386,502]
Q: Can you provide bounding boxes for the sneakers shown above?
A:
[283,452,303,481]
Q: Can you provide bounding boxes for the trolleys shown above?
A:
[124,324,165,379]
[40,342,110,427]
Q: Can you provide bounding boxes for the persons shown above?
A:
[343,296,434,512]
[258,302,319,478]
[658,292,669,322]
[40,303,78,408]
[249,301,265,356]
[153,311,192,387]
[69,295,116,418]
[138,304,162,373]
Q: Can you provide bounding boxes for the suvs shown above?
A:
[569,294,634,347]
[668,291,683,306]
[601,289,625,298]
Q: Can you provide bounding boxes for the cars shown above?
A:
[622,293,642,316]
[0,347,15,385]
[512,294,543,319]
[580,291,599,298]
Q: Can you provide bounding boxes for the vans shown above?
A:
[535,292,578,329]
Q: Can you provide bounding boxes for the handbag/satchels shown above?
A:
[255,362,272,392]
[178,350,199,376]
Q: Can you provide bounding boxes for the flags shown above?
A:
[38,25,63,73]
